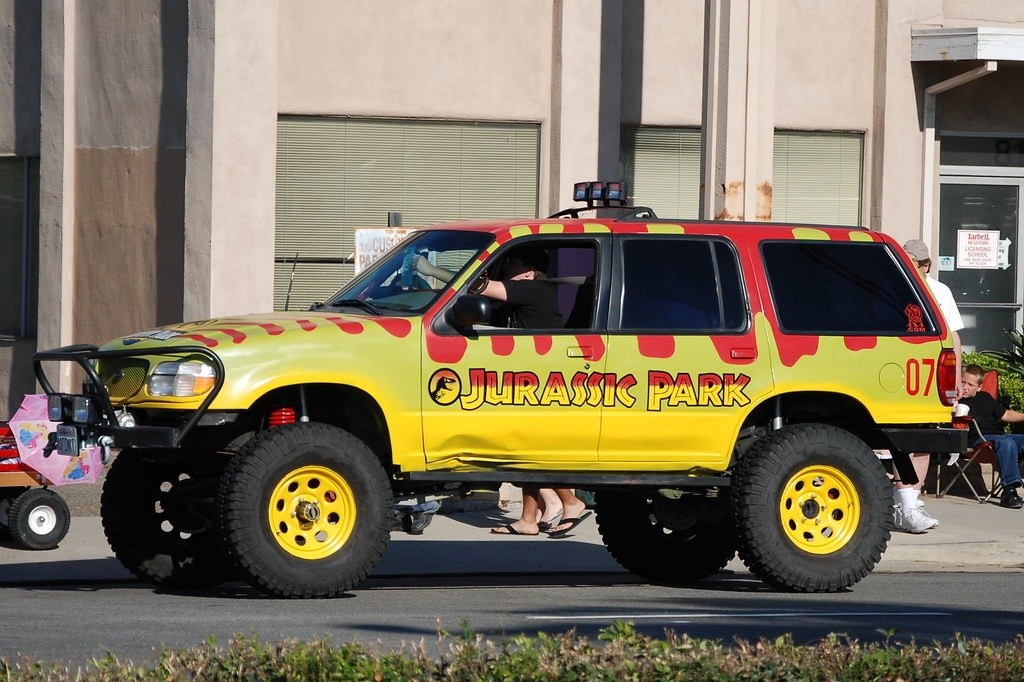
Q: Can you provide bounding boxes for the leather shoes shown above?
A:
[1001,488,1023,508]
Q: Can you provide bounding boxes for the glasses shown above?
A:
[915,261,927,268]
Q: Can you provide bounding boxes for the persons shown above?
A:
[409,248,563,330]
[393,484,592,539]
[958,364,1024,508]
[889,239,963,535]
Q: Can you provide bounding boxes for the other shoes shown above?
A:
[411,511,434,532]
[390,512,412,531]
[537,508,563,531]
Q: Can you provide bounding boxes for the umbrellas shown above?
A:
[7,393,109,486]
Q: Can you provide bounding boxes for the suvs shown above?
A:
[32,179,972,600]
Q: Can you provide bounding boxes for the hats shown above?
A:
[903,240,929,261]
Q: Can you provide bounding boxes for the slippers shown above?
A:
[491,523,539,535]
[549,511,592,536]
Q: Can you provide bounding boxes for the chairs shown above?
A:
[936,370,1024,505]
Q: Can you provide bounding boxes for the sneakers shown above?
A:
[894,504,939,533]
